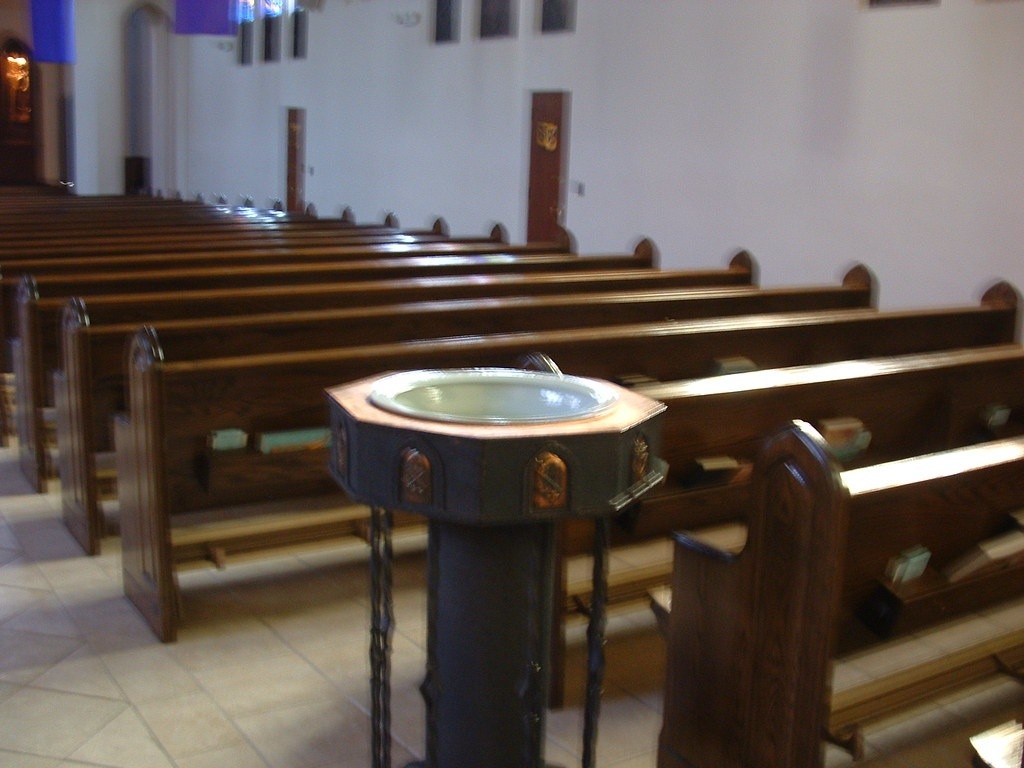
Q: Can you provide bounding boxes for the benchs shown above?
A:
[0,188,1024,768]
